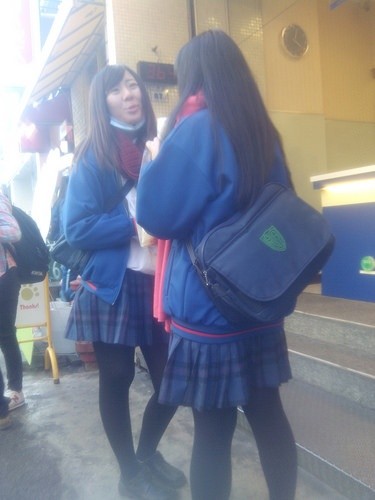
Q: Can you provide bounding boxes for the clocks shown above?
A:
[281,23,309,57]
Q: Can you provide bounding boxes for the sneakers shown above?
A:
[0,417,11,430]
[4,388,26,412]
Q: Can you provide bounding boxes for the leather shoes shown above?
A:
[136,448,188,488]
[119,465,182,500]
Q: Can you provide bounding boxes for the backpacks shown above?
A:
[1,204,50,284]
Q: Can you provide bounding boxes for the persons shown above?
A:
[133,29,301,500]
[0,183,27,413]
[0,369,12,431]
[60,64,190,499]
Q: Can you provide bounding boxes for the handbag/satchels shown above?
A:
[47,173,93,274]
[193,181,338,328]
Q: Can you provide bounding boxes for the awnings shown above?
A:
[14,0,105,127]
